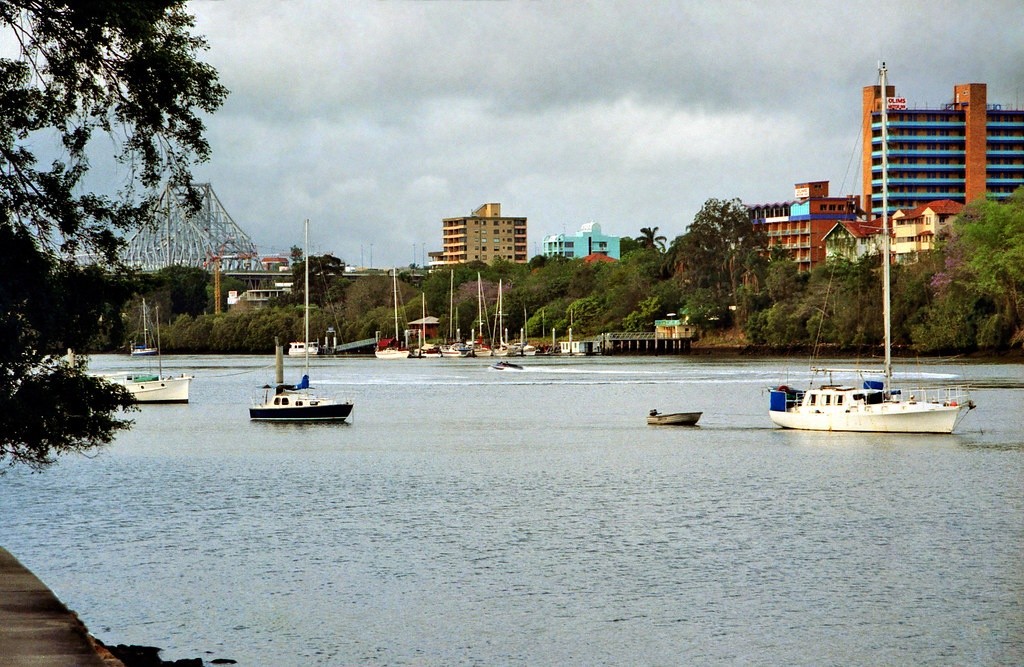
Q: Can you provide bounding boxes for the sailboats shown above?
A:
[763,61,979,435]
[489,278,514,356]
[437,268,473,358]
[374,266,412,358]
[509,306,538,357]
[289,294,322,355]
[469,271,493,356]
[101,306,192,404]
[132,297,160,356]
[248,218,356,424]
[408,291,443,359]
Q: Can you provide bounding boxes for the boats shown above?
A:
[647,408,705,426]
[492,361,525,372]
